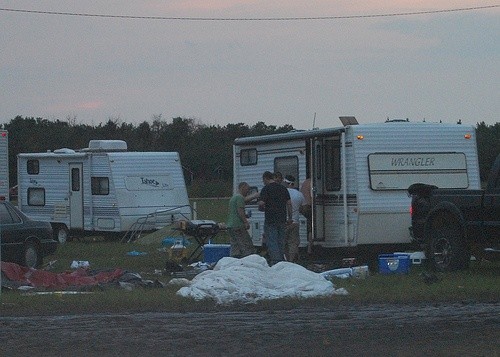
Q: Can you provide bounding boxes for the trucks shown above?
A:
[232,115,483,250]
[0,124,10,203]
[17,140,192,244]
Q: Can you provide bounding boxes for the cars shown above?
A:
[0,198,58,270]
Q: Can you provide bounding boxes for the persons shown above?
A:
[282,174,305,264]
[299,177,312,241]
[227,181,261,259]
[257,171,293,267]
[274,171,283,185]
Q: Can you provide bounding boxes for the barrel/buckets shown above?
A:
[168,242,188,262]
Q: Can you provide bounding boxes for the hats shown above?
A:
[283,175,296,183]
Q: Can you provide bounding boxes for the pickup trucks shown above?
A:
[406,158,500,274]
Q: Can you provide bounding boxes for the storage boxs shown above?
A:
[202,244,230,263]
[377,254,410,275]
